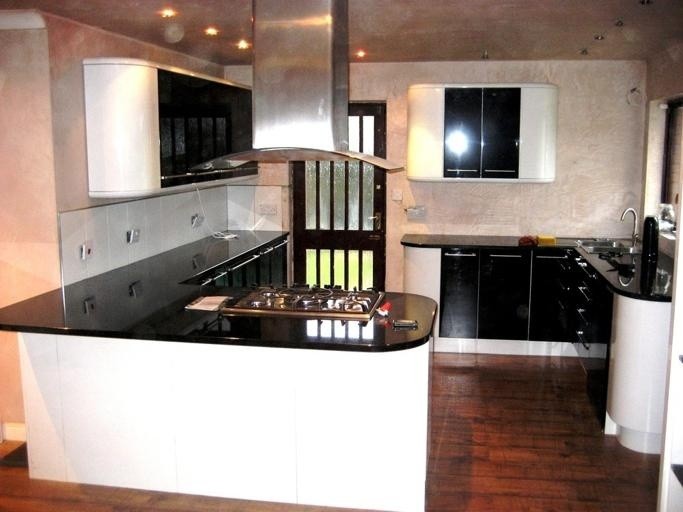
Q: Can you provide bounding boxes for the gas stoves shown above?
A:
[218,285,385,322]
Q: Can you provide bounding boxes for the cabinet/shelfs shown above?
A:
[200,270,228,290]
[230,255,260,287]
[408,81,556,186]
[404,247,529,356]
[568,249,621,435]
[259,241,288,287]
[83,57,252,201]
[530,248,573,356]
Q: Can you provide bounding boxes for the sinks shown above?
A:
[579,240,629,248]
[586,246,642,255]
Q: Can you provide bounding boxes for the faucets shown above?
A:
[621,208,640,247]
[619,256,636,287]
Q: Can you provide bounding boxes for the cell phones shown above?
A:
[392,319,419,328]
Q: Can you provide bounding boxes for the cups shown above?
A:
[653,270,670,296]
